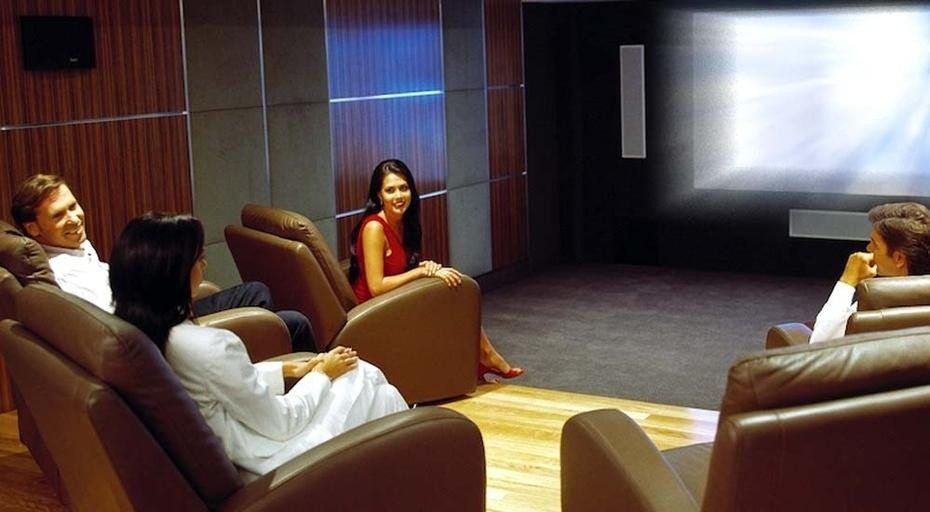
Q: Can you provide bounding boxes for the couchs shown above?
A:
[223,204,484,412]
[2,282,488,510]
[0,221,291,483]
[560,324,929,510]
[763,275,929,356]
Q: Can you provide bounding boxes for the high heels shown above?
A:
[479,357,526,384]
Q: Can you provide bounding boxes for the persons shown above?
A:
[114,210,417,482]
[349,159,526,391]
[806,199,930,345]
[9,168,321,355]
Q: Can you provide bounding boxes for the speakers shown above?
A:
[19,15,96,68]
[620,45,646,159]
[789,209,872,241]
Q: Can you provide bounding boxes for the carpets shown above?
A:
[480,260,833,410]
